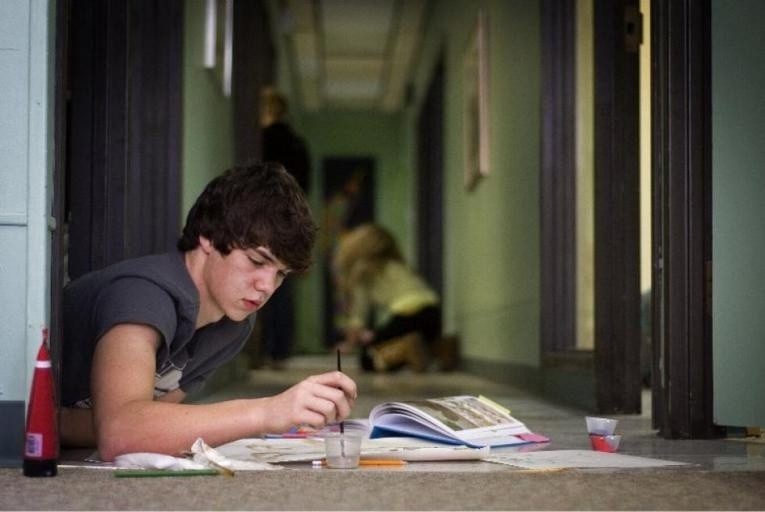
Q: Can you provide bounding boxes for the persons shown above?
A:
[56,160,357,463]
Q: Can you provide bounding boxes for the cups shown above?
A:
[591,433,623,452]
[324,430,362,468]
[585,415,619,434]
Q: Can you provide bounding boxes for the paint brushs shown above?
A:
[337,348,346,458]
[181,450,235,477]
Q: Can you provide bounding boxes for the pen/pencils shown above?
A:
[114,469,220,478]
[312,460,408,465]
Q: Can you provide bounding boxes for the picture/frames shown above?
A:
[459,8,488,192]
[204,0,234,99]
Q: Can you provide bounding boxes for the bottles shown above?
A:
[20,329,59,478]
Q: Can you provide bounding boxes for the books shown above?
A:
[261,395,551,448]
